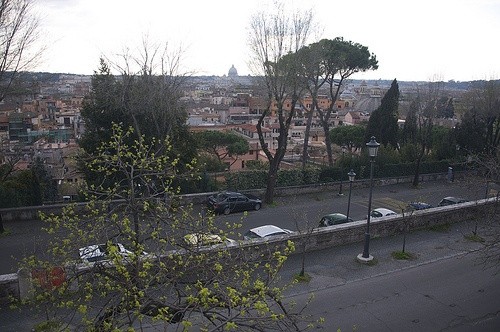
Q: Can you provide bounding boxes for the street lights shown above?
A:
[452,144,461,183]
[361,136,380,258]
[346,169,356,223]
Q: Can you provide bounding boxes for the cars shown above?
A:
[405,202,434,212]
[365,207,396,220]
[78,242,138,262]
[206,191,262,215]
[319,212,354,227]
[243,225,294,241]
[437,196,469,208]
[183,235,237,247]
